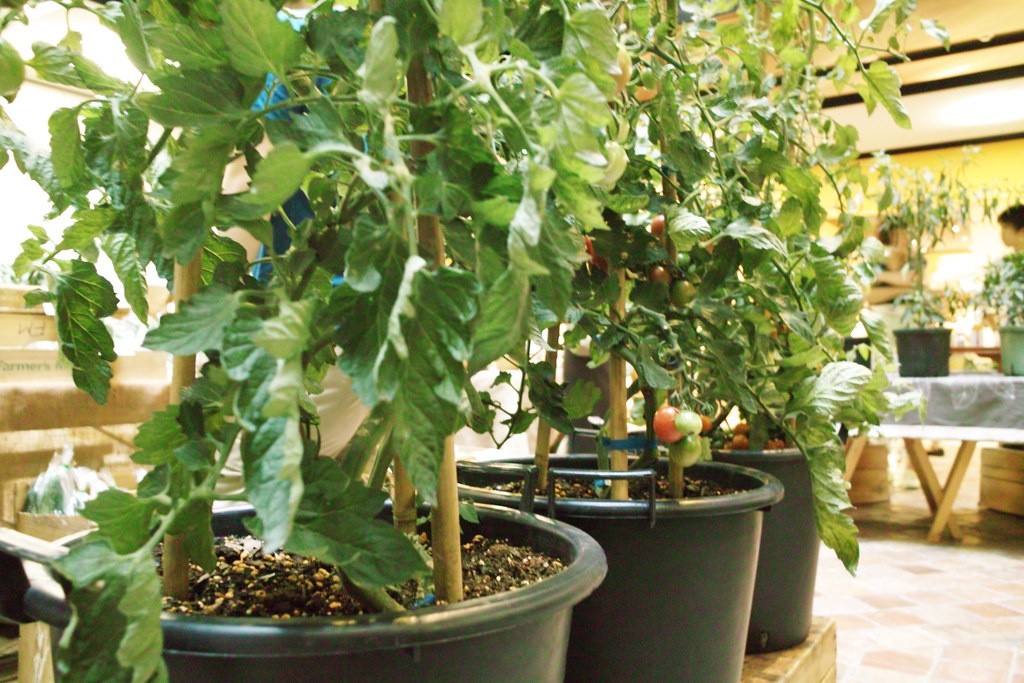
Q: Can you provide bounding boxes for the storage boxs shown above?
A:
[981,445,1024,515]
[840,438,889,504]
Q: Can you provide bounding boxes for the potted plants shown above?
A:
[0,0,956,683]
[866,148,998,378]
[980,253,1024,375]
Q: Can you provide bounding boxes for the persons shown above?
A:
[211,1,411,497]
[853,214,944,455]
[998,203,1024,252]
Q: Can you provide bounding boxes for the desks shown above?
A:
[838,375,1024,544]
